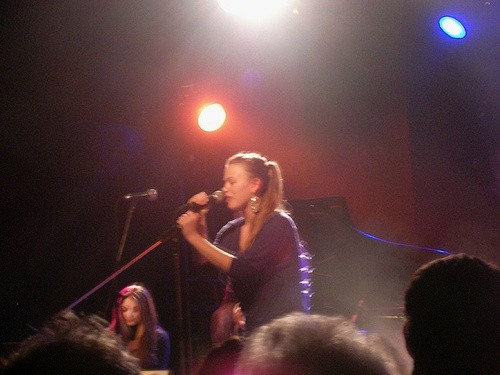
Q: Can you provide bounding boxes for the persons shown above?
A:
[351,298,370,331]
[110,283,171,375]
[223,302,247,349]
[404,252,500,375]
[5,315,139,375]
[175,151,309,350]
[236,312,407,375]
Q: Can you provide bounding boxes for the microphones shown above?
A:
[351,300,365,325]
[123,189,158,201]
[178,191,224,215]
